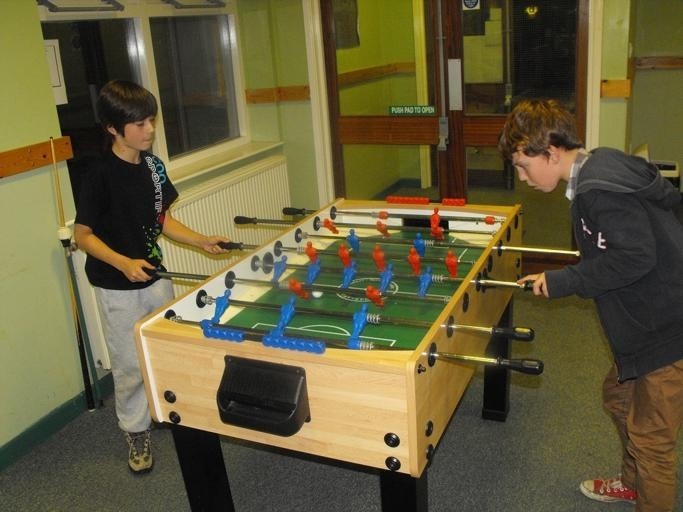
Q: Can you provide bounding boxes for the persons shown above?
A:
[499,100,683,512]
[71,78,232,472]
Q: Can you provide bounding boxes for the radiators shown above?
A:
[71,151,291,373]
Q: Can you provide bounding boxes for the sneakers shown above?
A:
[580,477,637,506]
[125,430,153,474]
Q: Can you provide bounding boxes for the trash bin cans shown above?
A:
[631,144,680,187]
